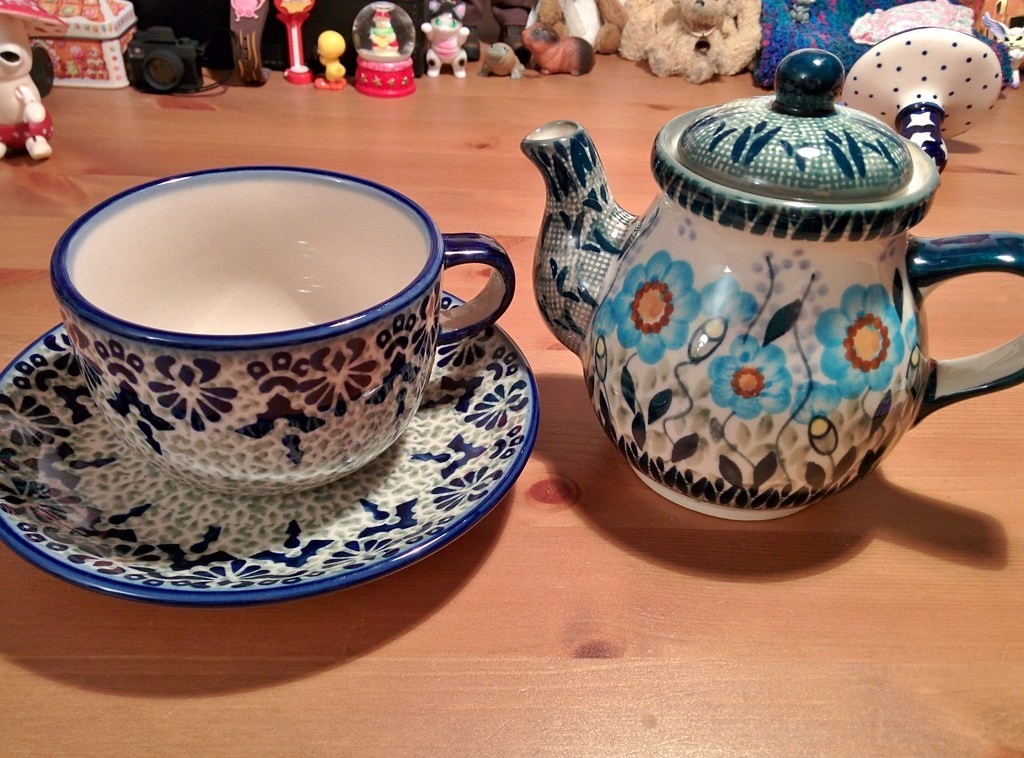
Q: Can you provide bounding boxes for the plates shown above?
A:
[0,288,540,606]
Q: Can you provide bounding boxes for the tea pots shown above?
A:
[518,46,1024,523]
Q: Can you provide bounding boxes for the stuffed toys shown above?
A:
[524,0,763,87]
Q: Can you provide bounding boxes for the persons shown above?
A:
[457,0,531,65]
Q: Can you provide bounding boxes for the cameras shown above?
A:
[121,28,204,96]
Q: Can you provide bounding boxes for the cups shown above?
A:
[49,164,518,499]
